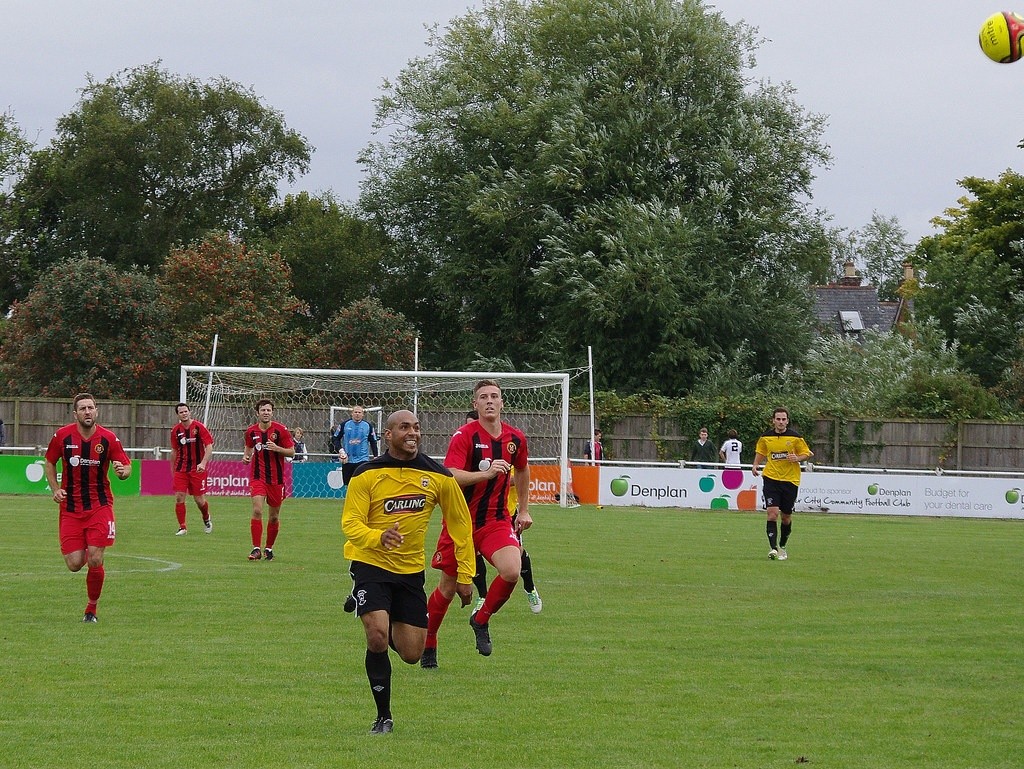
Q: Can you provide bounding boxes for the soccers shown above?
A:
[978,11,1024,64]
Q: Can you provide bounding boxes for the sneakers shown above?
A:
[472,598,485,615]
[203,515,212,534]
[343,595,356,612]
[778,545,787,560]
[420,648,437,669]
[176,528,187,535]
[371,717,393,733]
[769,550,776,560]
[524,586,542,613]
[249,548,261,559]
[265,550,274,561]
[83,612,97,622]
[470,610,492,656]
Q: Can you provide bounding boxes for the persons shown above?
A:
[242,400,294,562]
[328,425,342,463]
[45,393,131,622]
[584,429,604,465]
[690,427,717,469]
[752,407,811,561]
[0,419,6,455]
[333,406,378,485]
[466,411,542,618]
[171,403,213,536]
[719,429,742,470]
[341,409,476,735]
[286,427,308,462]
[418,381,533,673]
